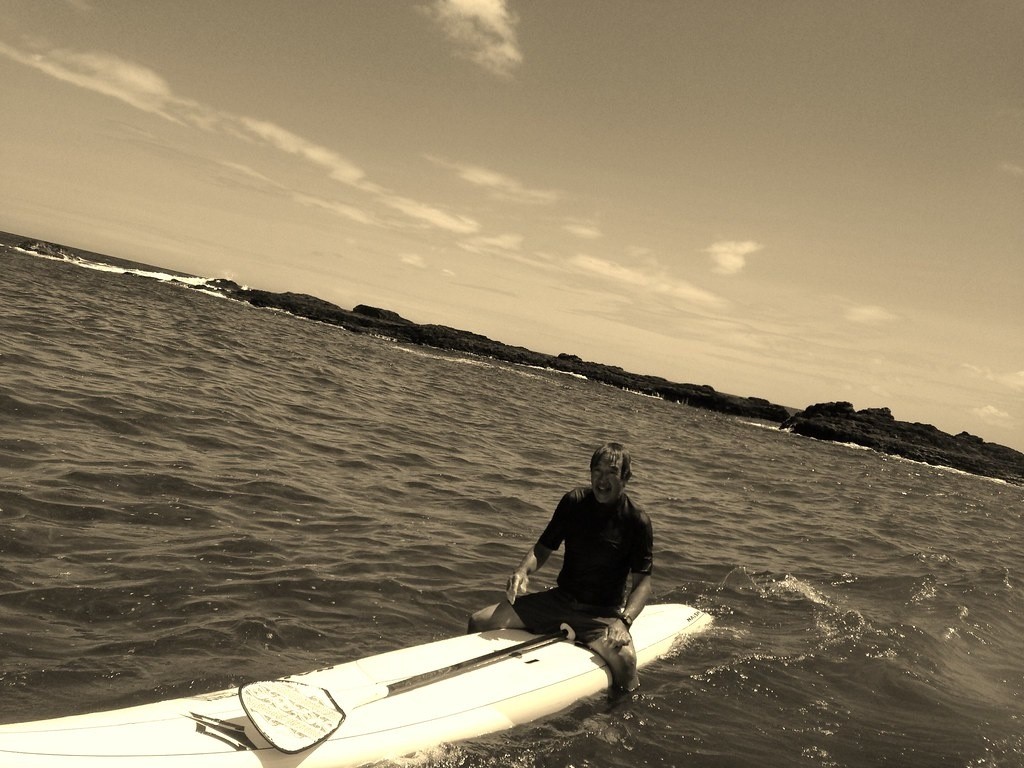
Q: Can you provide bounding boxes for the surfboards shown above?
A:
[2,600,715,768]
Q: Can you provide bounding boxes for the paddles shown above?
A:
[238,621,578,756]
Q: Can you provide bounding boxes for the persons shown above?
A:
[467,442,653,697]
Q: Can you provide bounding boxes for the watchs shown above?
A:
[619,614,632,628]
